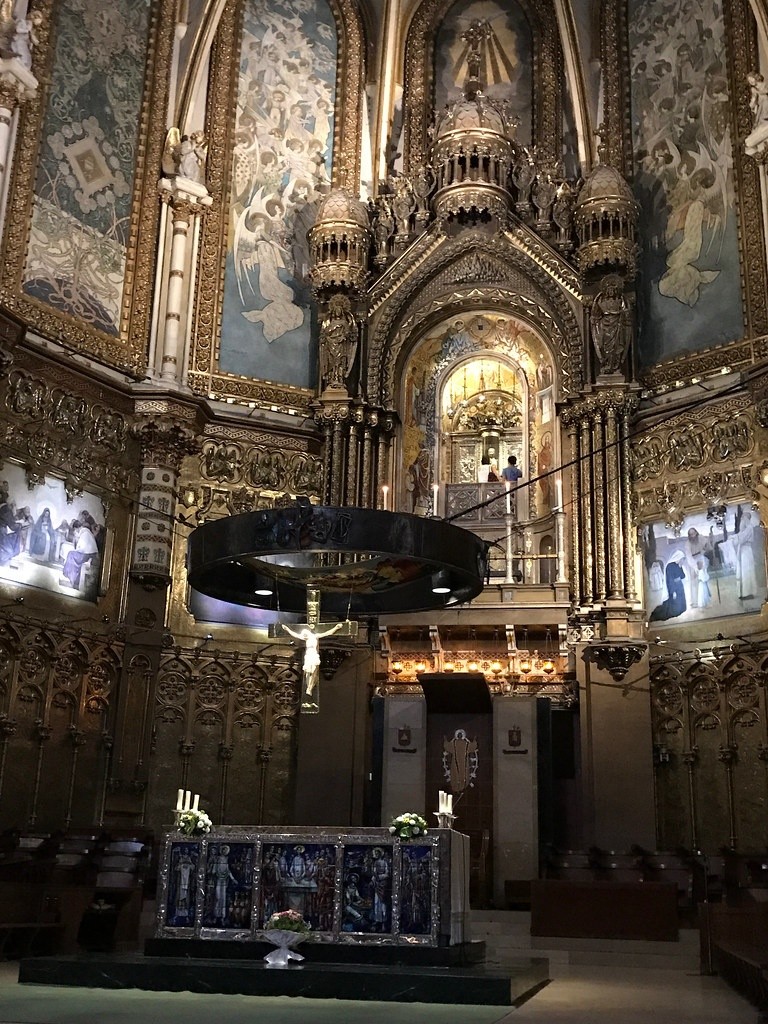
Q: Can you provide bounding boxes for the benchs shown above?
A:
[708,899,768,1014]
[531,878,679,942]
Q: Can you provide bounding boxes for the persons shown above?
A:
[488,448,499,471]
[281,623,343,697]
[478,455,500,482]
[501,456,522,481]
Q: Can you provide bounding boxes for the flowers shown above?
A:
[263,910,311,966]
[389,812,428,837]
[177,810,212,836]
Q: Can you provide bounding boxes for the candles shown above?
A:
[439,790,452,814]
[177,789,184,811]
[184,791,191,810]
[193,795,199,810]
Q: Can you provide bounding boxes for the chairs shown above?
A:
[0,830,152,887]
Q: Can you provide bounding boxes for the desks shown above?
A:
[0,923,61,953]
